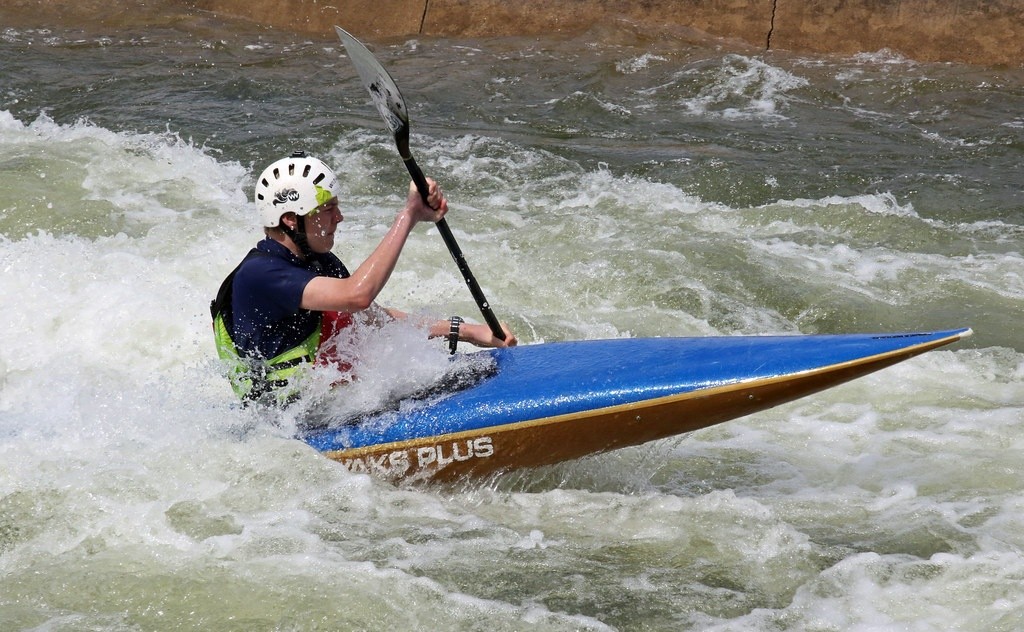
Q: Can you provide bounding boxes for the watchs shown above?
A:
[446,313,467,356]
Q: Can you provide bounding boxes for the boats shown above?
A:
[297,326,973,496]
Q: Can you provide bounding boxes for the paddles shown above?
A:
[333,22,508,343]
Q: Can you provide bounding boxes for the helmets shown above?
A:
[254,153,341,226]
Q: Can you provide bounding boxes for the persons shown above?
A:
[209,146,517,411]
